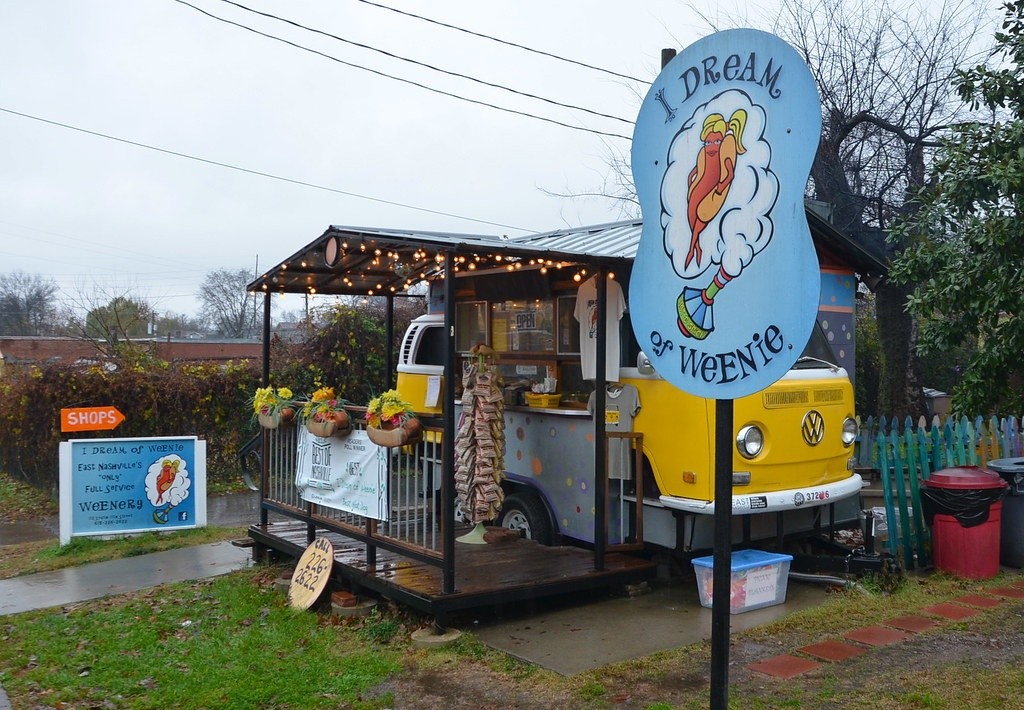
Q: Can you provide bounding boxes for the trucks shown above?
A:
[395,257,861,565]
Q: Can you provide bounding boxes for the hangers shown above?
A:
[607,381,623,391]
[590,272,599,280]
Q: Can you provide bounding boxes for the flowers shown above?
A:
[301,389,349,421]
[365,389,415,429]
[252,384,292,414]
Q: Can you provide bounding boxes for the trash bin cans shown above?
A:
[986,457,1024,570]
[918,465,1008,581]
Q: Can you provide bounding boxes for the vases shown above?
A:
[366,418,422,447]
[308,413,346,438]
[259,409,294,430]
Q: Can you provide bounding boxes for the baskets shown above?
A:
[525,392,561,408]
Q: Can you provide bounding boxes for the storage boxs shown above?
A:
[691,550,794,614]
[526,394,561,408]
